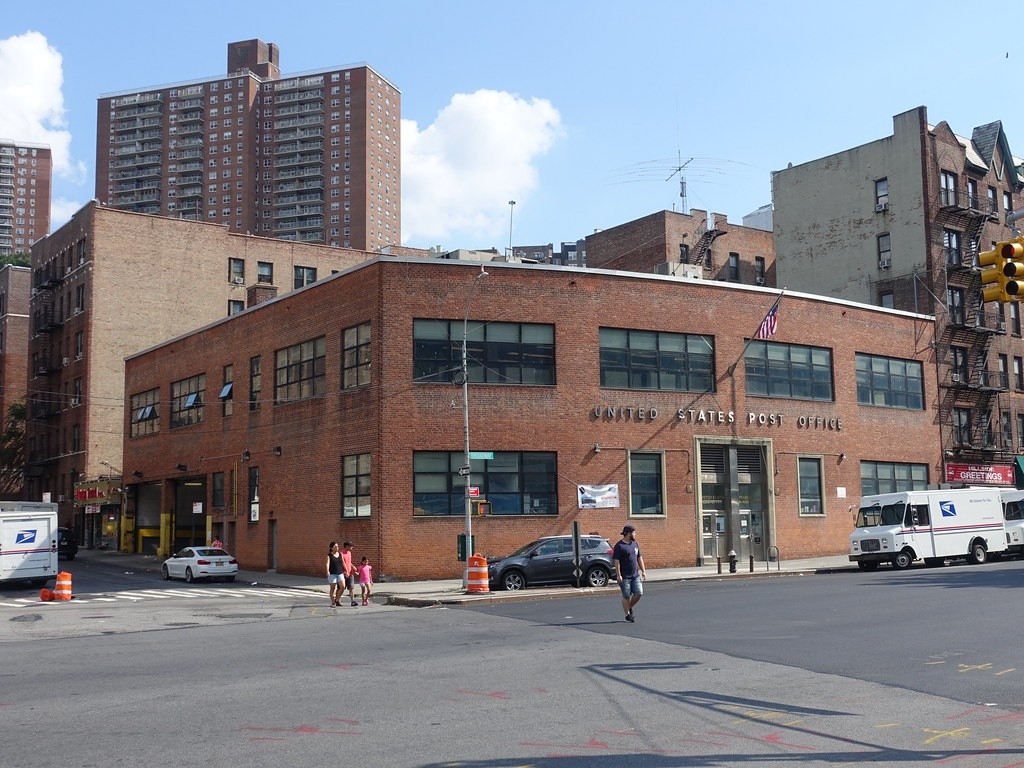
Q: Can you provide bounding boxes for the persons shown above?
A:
[354,556,373,606]
[212,536,224,549]
[612,525,646,623]
[325,542,345,608]
[336,541,359,607]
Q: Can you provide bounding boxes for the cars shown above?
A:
[160,547,238,583]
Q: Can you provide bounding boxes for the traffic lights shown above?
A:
[1001,236,1024,301]
[978,242,1000,304]
[477,500,493,516]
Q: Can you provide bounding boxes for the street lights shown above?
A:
[463,270,488,588]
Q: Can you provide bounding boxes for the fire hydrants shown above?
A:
[728,550,738,572]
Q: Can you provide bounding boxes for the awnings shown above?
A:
[1014,456,1024,489]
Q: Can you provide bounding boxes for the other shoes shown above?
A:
[625,614,634,622]
[365,599,369,604]
[334,599,343,606]
[362,601,367,605]
[351,601,358,606]
[331,604,336,607]
[629,607,633,616]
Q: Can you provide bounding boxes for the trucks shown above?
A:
[1001,490,1024,557]
[849,489,1008,570]
[0,501,59,585]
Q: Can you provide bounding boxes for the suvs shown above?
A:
[486,535,617,592]
[58,527,78,559]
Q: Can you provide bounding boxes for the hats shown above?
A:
[344,540,354,547]
[620,525,635,535]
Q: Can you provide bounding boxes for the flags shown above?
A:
[757,304,779,339]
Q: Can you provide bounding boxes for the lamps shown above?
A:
[176,463,187,472]
[271,447,281,458]
[241,452,250,462]
[132,470,142,478]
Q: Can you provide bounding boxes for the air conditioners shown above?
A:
[74,308,82,313]
[78,257,84,268]
[32,287,37,295]
[236,276,243,284]
[66,267,72,273]
[70,397,78,407]
[991,211,999,218]
[876,203,888,212]
[953,373,962,382]
[63,357,71,366]
[878,260,891,267]
[757,276,765,283]
[78,351,83,358]
